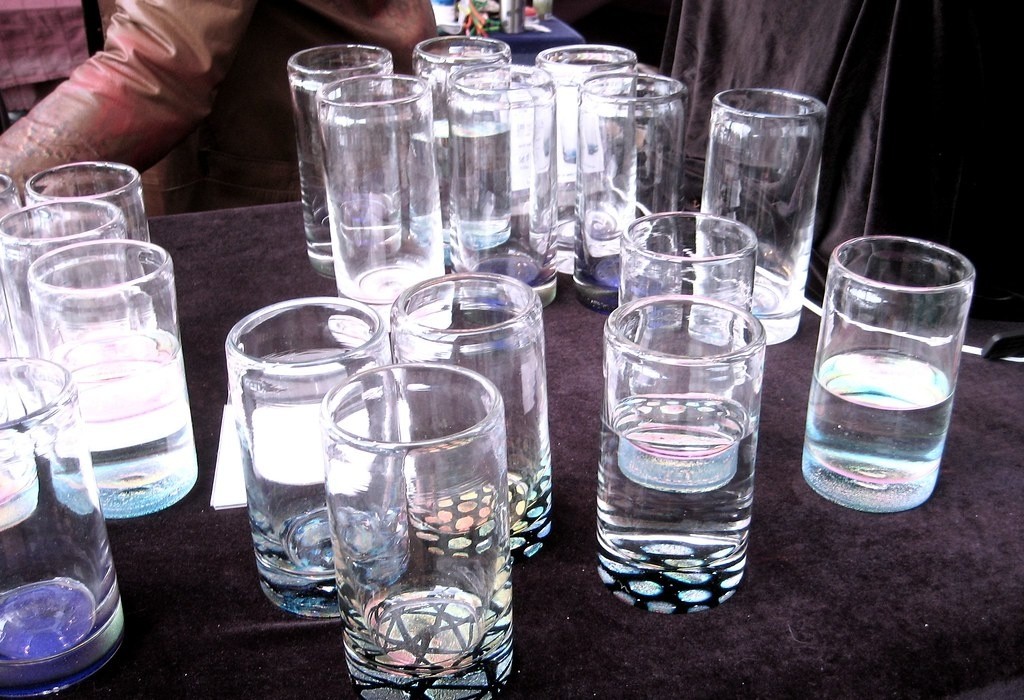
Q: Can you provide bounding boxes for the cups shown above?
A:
[0,174,23,222]
[25,239,197,520]
[802,236,975,513]
[24,161,159,330]
[597,297,766,615]
[289,36,686,314]
[0,356,125,698]
[430,0,456,26]
[0,201,137,453]
[690,89,827,347]
[532,0,553,22]
[388,275,555,562]
[618,212,757,493]
[0,309,40,532]
[225,295,411,620]
[322,362,513,699]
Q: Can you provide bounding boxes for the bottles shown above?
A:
[498,0,525,34]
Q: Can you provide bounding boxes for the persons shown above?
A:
[0,0,437,213]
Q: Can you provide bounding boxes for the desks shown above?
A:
[0,202,1024,700]
